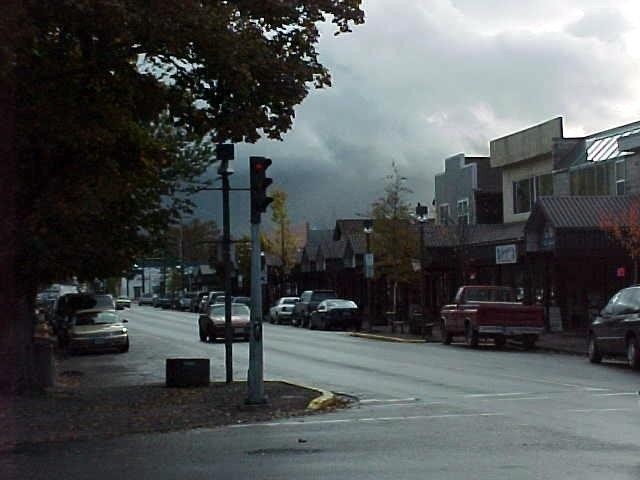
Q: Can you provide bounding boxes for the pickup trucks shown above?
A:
[438,284,545,349]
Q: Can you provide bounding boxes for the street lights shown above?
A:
[363,219,375,332]
[134,255,146,293]
[415,202,429,339]
[211,140,238,384]
[176,262,187,298]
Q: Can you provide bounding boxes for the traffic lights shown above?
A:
[250,155,275,215]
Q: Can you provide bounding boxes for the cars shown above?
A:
[267,288,365,333]
[37,284,130,353]
[585,284,640,368]
[136,291,252,344]
